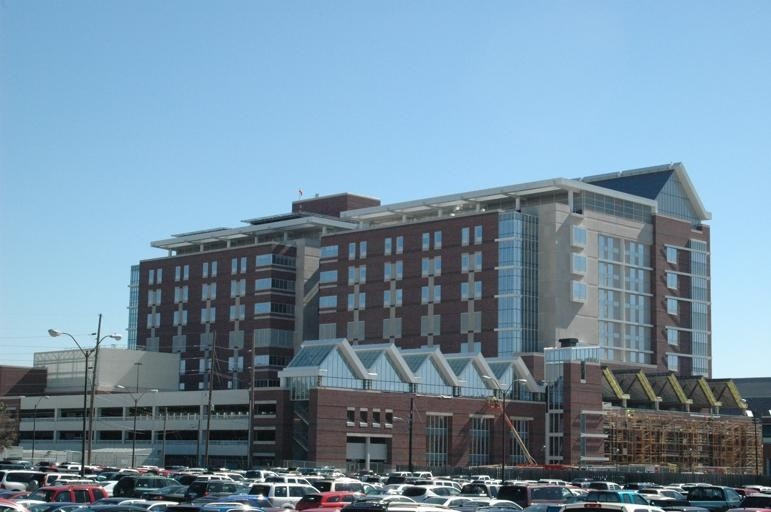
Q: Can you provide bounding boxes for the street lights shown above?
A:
[48,327,122,477]
[114,384,159,467]
[740,398,771,482]
[19,394,50,461]
[481,374,528,482]
